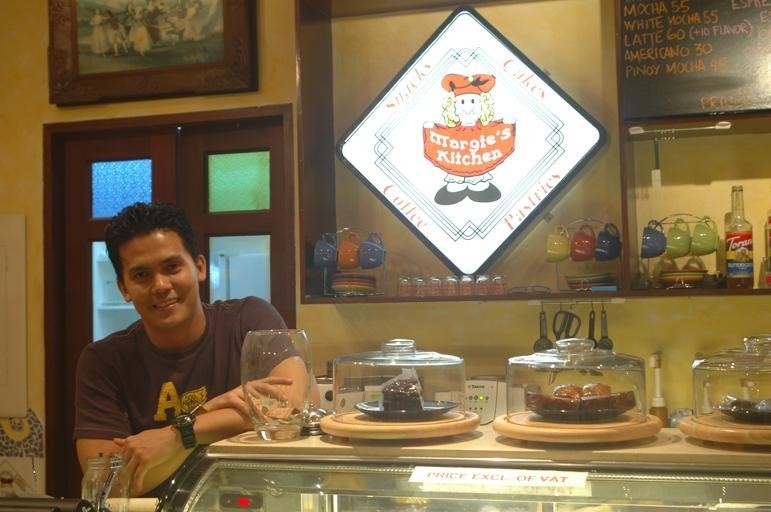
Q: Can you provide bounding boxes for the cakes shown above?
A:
[382,376,424,411]
[712,393,770,425]
[526,379,638,422]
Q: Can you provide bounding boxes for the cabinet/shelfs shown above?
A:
[156,426,771,511]
[293,1,770,304]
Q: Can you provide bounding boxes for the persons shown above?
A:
[89,2,202,58]
[71,201,321,499]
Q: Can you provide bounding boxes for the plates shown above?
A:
[534,408,622,423]
[357,399,461,420]
[710,402,770,426]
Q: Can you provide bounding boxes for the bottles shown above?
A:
[723,184,754,290]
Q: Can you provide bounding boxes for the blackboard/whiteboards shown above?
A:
[616,2,770,118]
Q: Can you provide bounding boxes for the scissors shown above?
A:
[548,311,581,384]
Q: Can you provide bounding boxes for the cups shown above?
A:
[568,223,597,260]
[336,233,361,269]
[690,217,720,254]
[639,219,666,259]
[358,231,387,267]
[392,273,509,297]
[662,217,693,259]
[78,455,132,512]
[543,224,573,262]
[311,231,339,266]
[593,223,622,259]
[239,325,313,445]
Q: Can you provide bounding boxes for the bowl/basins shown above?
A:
[659,269,709,286]
[566,272,612,289]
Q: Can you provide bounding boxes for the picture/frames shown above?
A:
[47,0,257,104]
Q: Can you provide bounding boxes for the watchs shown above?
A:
[172,412,196,450]
